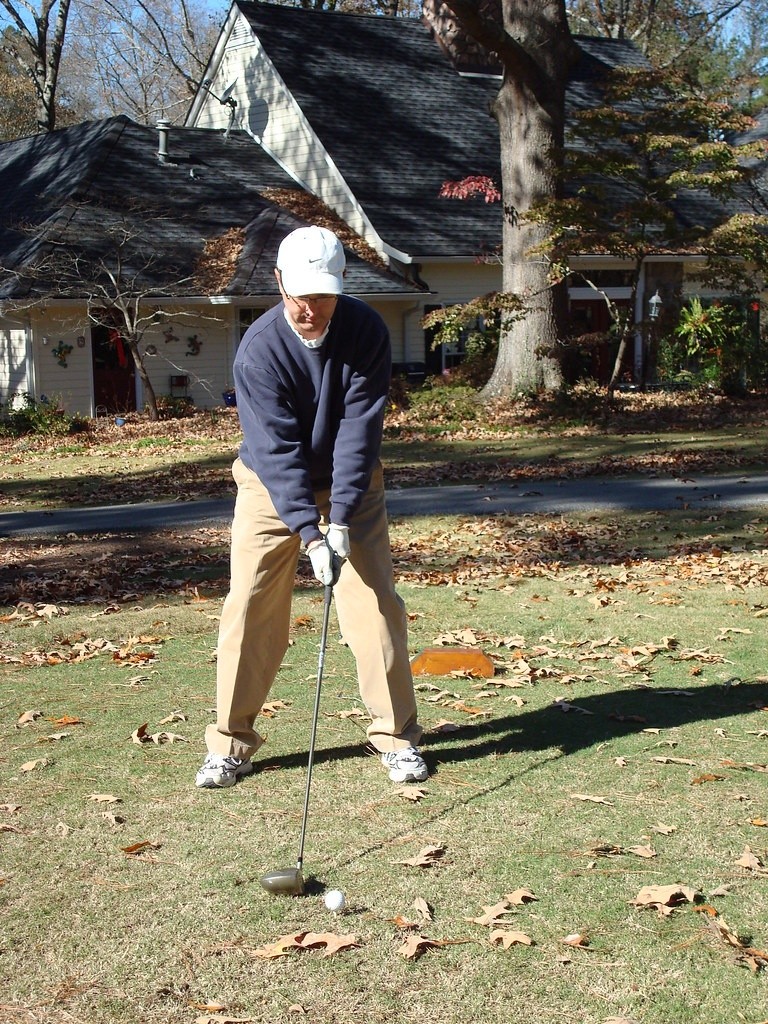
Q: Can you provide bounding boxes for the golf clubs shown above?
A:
[258,586,332,893]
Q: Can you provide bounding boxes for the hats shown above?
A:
[278,226,345,296]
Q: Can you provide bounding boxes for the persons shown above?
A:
[193,224,428,789]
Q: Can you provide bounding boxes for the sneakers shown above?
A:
[381,746,428,782]
[196,753,254,787]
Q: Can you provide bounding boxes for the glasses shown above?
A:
[280,281,337,305]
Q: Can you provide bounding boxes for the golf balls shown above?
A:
[325,890,344,910]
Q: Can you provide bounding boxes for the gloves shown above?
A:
[324,524,351,560]
[305,539,343,586]
